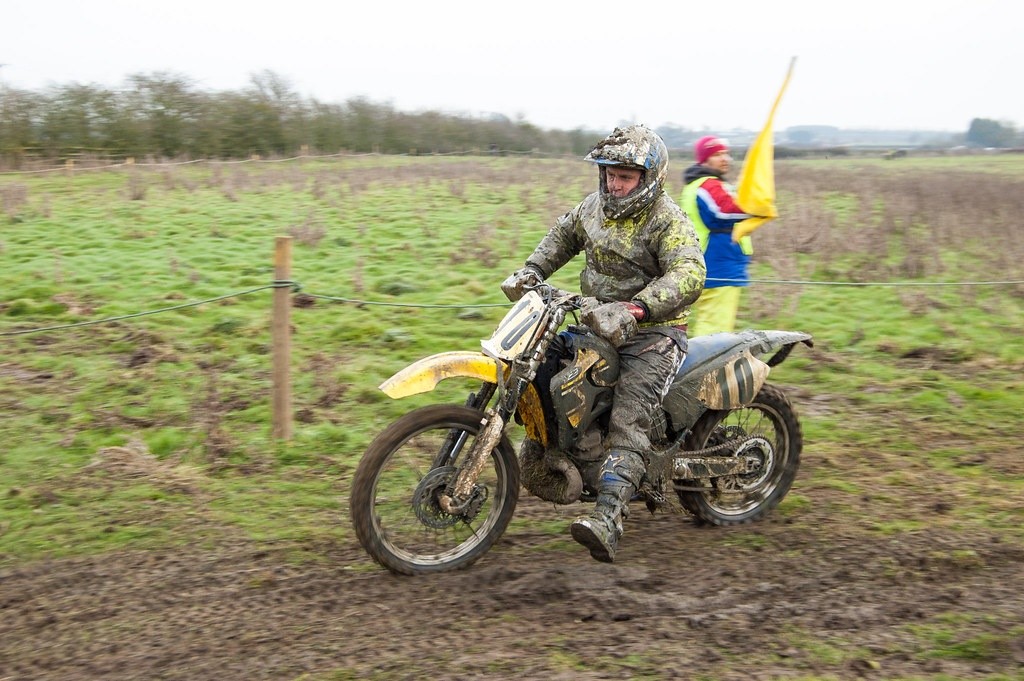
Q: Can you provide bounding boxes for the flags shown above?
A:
[731,122,779,240]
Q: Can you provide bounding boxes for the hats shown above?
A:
[694,135,727,164]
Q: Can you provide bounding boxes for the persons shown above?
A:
[500,126,706,563]
[682,135,754,337]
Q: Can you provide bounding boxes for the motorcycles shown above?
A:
[346,289,816,579]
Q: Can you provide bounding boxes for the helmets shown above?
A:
[583,125,670,220]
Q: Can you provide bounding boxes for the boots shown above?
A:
[569,450,647,563]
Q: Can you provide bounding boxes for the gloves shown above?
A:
[622,301,644,320]
